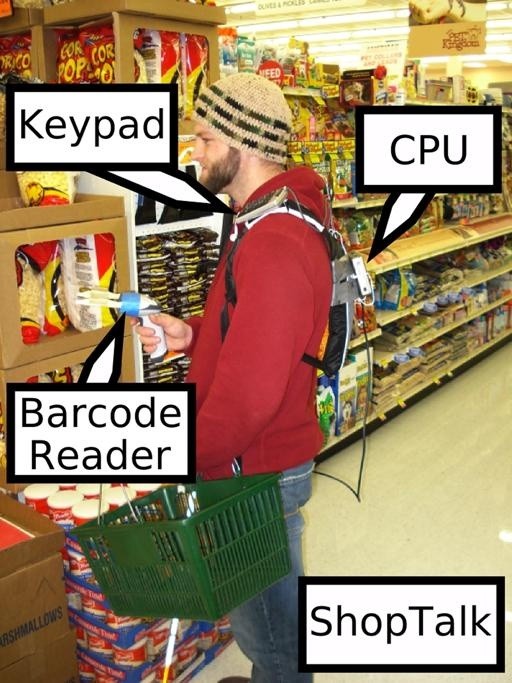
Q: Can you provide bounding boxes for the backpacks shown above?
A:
[225,200,360,383]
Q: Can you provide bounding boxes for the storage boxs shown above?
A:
[1,488,79,682]
[0,192,135,371]
[72,474,297,623]
[3,339,138,451]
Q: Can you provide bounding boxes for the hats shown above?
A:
[191,69,293,167]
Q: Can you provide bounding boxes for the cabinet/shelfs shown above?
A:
[293,91,512,462]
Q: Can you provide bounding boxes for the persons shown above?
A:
[124,69,341,683]
